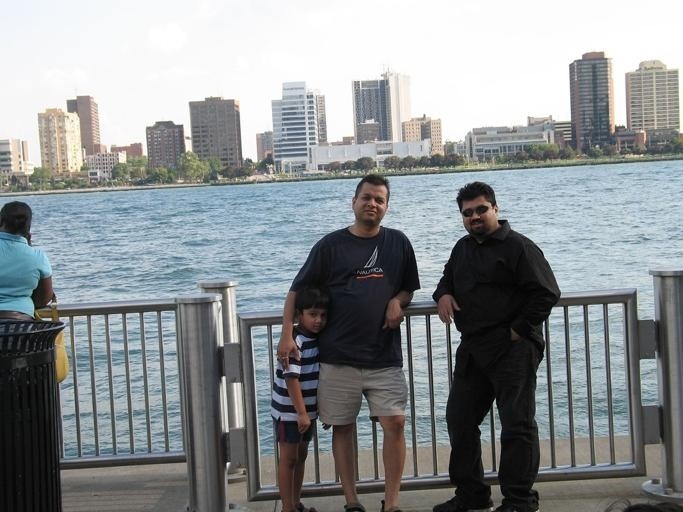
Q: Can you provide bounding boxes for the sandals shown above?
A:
[381,500,402,512]
[297,503,317,512]
[344,503,365,511]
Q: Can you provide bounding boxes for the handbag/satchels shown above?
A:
[34,293,69,384]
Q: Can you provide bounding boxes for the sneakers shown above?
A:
[433,495,494,512]
[494,502,539,512]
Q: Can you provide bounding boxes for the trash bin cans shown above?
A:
[0,320,65,512]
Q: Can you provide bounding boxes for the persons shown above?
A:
[433,181,561,512]
[0,200,54,320]
[269,286,330,511]
[276,173,420,511]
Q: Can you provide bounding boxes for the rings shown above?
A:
[282,357,288,360]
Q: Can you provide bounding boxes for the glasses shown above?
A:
[463,205,488,217]
